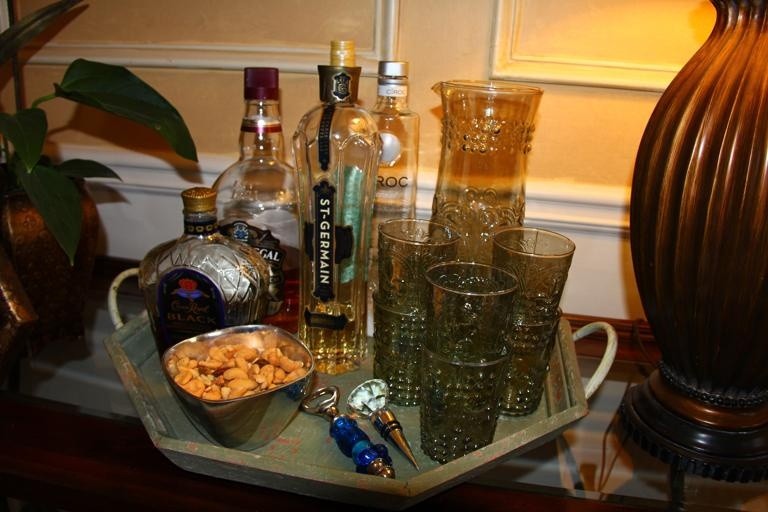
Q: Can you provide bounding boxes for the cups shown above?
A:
[375,78,578,464]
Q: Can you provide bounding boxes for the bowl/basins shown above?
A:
[158,325,316,453]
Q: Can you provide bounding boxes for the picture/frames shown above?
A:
[488,0,683,93]
[0,0,403,78]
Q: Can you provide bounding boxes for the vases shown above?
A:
[620,0,768,483]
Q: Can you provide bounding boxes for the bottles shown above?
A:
[138,35,422,378]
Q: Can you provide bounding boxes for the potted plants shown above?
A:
[0,55,201,362]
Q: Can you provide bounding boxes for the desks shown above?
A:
[0,297,768,512]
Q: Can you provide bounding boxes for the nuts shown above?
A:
[167,329,308,401]
